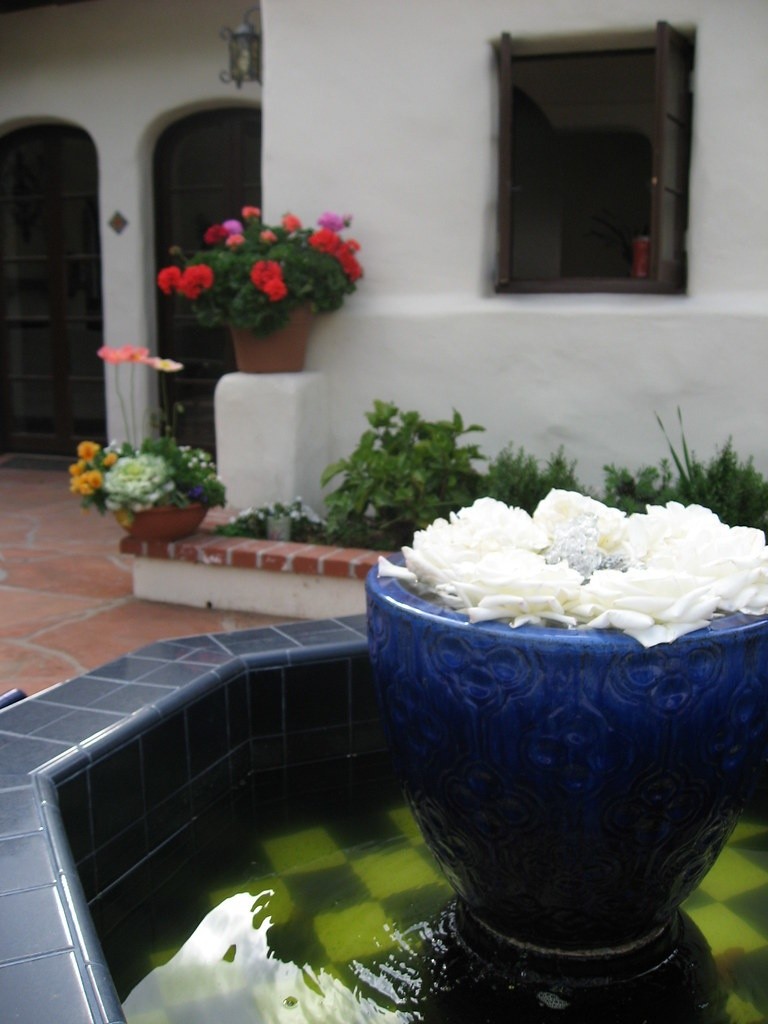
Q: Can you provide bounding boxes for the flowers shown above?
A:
[67,344,230,527]
[365,485,768,650]
[159,203,368,338]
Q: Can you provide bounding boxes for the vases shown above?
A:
[361,553,768,1024]
[122,501,209,539]
[231,302,316,371]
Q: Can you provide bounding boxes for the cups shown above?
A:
[632,241,649,278]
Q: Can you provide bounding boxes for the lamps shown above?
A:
[219,5,262,90]
